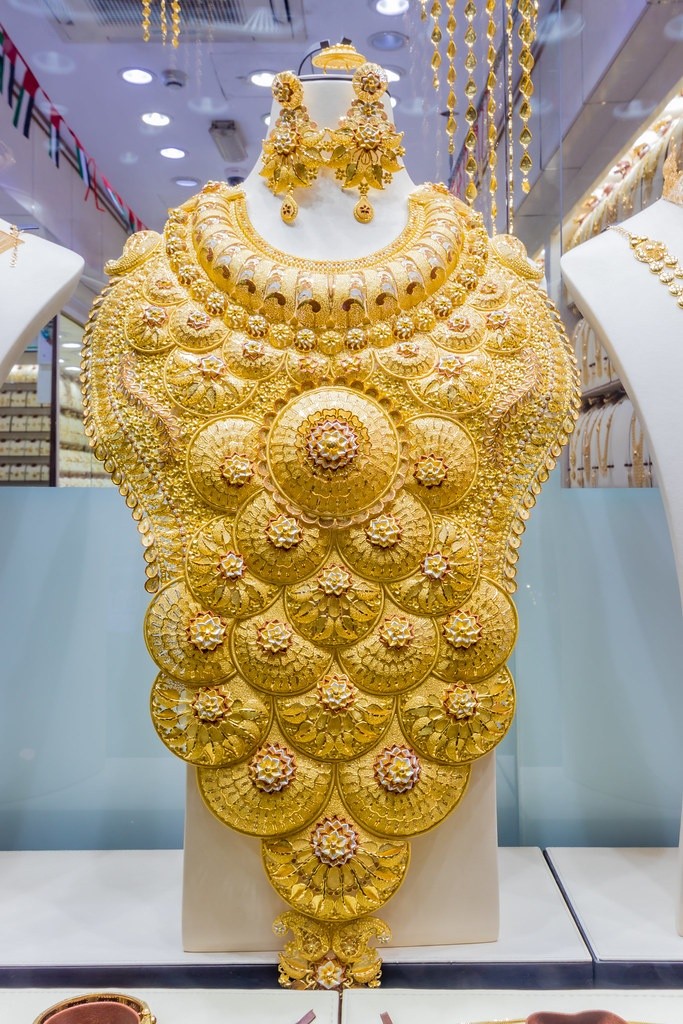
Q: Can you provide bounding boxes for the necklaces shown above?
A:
[566,284,662,488]
[563,122,663,256]
[606,225,683,308]
